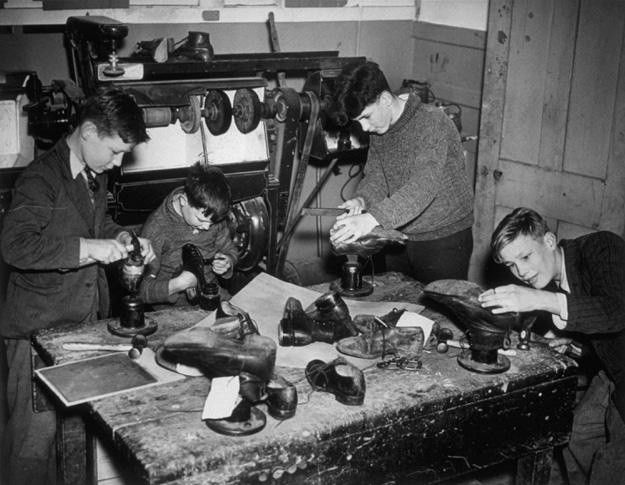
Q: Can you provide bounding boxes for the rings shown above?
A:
[492,287,496,295]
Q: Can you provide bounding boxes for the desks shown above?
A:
[31,270,585,485]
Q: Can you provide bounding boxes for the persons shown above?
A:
[0,88,152,484]
[327,59,474,284]
[480,208,624,483]
[134,161,239,309]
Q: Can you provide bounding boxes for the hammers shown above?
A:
[437,328,515,356]
[63,333,148,358]
[517,312,568,354]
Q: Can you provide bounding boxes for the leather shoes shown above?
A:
[353,307,407,335]
[329,230,389,257]
[266,374,297,421]
[163,328,277,387]
[336,326,425,359]
[306,357,365,406]
[216,301,260,340]
[182,244,204,306]
[118,229,144,291]
[424,278,518,335]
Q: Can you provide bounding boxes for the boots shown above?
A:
[137,37,174,63]
[303,293,358,336]
[278,297,336,347]
[170,32,213,62]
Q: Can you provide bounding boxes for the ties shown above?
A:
[84,165,97,191]
